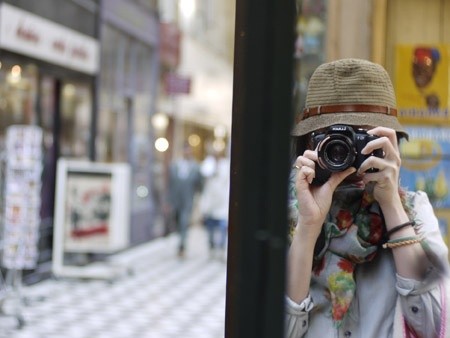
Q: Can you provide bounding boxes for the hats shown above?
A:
[290,59,409,142]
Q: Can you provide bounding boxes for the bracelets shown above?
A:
[383,220,423,248]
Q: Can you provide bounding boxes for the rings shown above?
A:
[292,164,302,169]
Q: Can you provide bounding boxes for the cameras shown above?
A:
[312,125,384,191]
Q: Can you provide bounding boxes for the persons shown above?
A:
[165,142,231,264]
[283,58,450,338]
